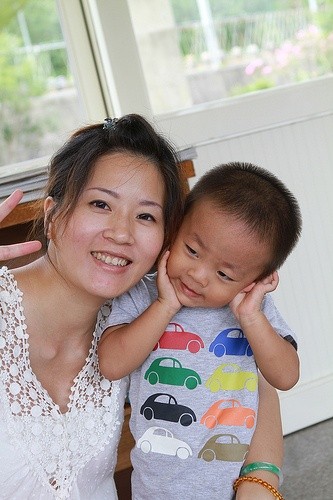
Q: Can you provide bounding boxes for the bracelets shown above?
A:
[239,462,284,489]
[233,475,284,500]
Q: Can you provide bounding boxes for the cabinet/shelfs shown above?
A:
[0,145,198,477]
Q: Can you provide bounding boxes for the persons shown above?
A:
[97,162,303,500]
[0,114,284,500]
